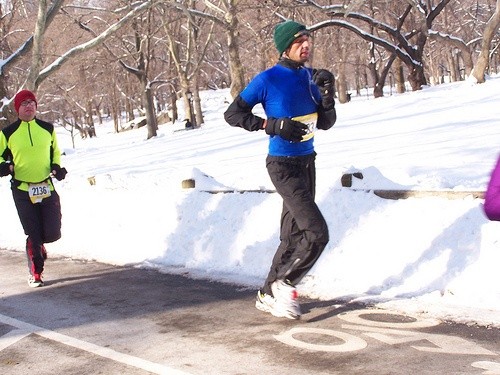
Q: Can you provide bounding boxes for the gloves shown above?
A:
[51,163,67,181]
[265,117,309,142]
[313,68,335,109]
[0,160,14,177]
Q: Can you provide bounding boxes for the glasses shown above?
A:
[292,38,309,44]
[21,99,36,106]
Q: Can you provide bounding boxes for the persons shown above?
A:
[0,90,67,288]
[223,21,337,321]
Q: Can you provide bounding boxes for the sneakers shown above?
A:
[256,289,298,320]
[41,245,48,260]
[28,273,44,288]
[271,279,302,317]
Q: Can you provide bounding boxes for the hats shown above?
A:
[14,90,38,113]
[274,20,309,57]
